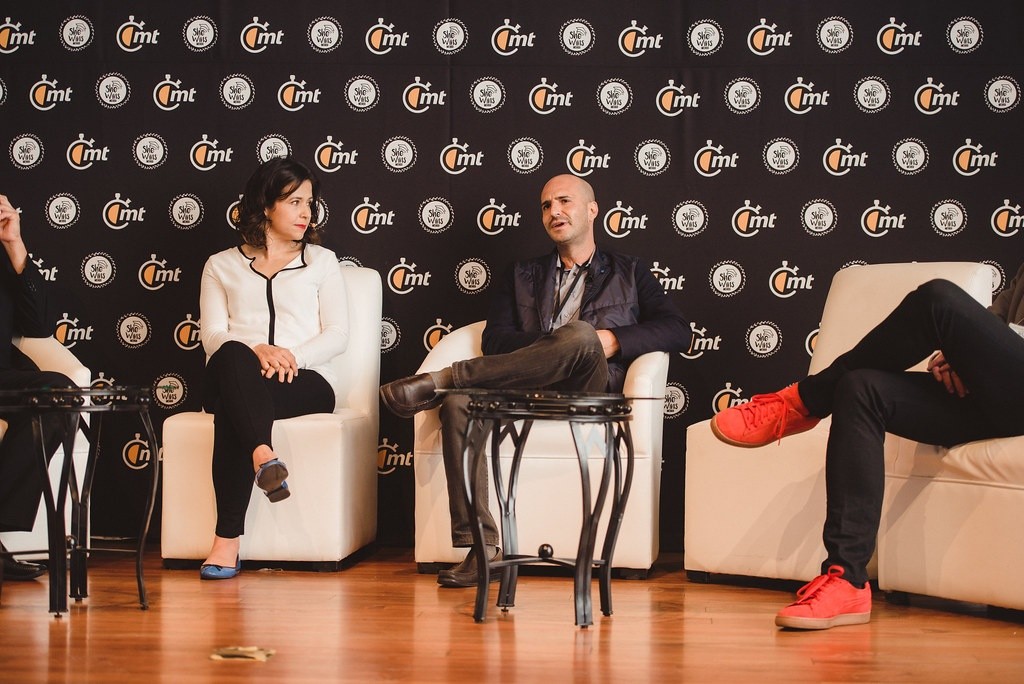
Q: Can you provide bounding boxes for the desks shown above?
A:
[467,394,638,629]
[15,386,160,618]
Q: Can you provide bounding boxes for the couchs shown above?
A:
[0,334,92,559]
[877,410,1024,610]
[684,263,993,584]
[161,267,383,572]
[413,319,670,575]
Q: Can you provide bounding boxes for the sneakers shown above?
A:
[710,388,821,447]
[775,565,872,629]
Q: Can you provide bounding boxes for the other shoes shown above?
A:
[256,456,290,502]
[200,548,241,579]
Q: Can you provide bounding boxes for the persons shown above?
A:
[199,158,351,579]
[711,263,1023,629]
[382,173,692,586]
[0,193,78,582]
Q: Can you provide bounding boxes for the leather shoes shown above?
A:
[380,371,444,418]
[0,552,47,580]
[436,545,503,587]
